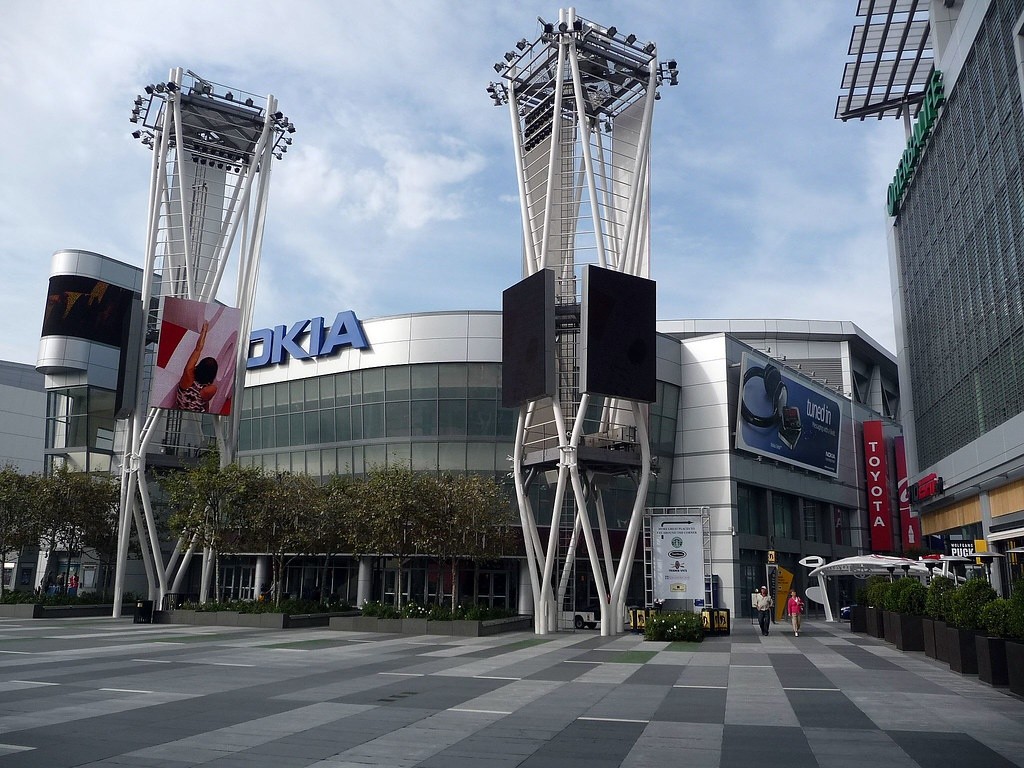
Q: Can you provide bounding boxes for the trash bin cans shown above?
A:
[134,600,153,624]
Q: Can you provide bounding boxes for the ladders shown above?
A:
[555,480,576,633]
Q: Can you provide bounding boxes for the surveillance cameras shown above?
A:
[732,531,736,537]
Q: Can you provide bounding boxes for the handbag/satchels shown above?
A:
[800,604,805,615]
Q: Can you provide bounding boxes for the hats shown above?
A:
[762,586,767,589]
[61,571,65,574]
[73,572,77,576]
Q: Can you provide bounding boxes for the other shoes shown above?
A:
[765,634,768,636]
[794,632,799,637]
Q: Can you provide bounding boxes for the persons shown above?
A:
[654,599,665,615]
[754,586,773,636]
[177,320,218,413]
[787,589,804,637]
[40,571,79,596]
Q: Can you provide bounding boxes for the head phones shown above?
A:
[741,363,788,428]
